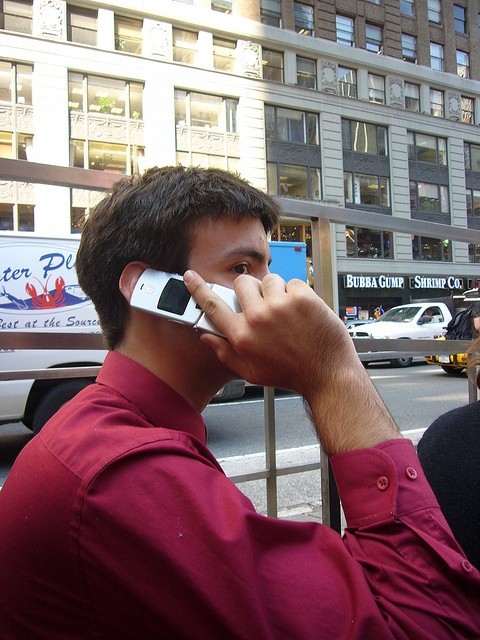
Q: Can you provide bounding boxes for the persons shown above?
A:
[424,309,440,324]
[468,302,480,336]
[1,164,480,640]
[417,337,480,535]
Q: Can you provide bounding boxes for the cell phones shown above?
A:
[128,267,243,339]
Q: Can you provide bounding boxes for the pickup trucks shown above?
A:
[346,303,453,367]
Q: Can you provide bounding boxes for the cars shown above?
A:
[424,325,480,372]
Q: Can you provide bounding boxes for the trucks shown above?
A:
[211,242,309,401]
[0,230,111,436]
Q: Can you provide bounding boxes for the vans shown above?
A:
[341,318,374,329]
